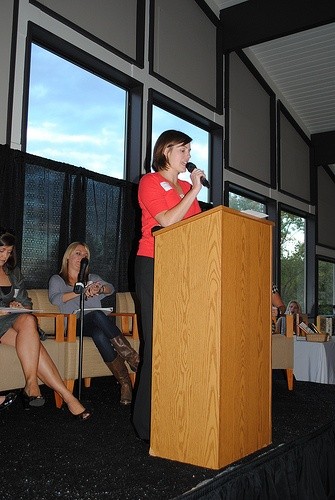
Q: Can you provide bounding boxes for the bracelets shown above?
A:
[98,285,105,295]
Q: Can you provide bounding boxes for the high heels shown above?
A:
[22,388,45,413]
[65,396,91,421]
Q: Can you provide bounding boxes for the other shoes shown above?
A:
[0,393,17,410]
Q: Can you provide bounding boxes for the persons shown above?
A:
[49,242,140,404]
[0,228,90,420]
[131,129,206,446]
[0,393,17,412]
[271,282,306,335]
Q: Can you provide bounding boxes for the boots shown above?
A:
[110,333,140,373]
[104,353,132,403]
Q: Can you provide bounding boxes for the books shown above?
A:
[0,307,42,312]
[296,321,321,334]
[73,307,113,319]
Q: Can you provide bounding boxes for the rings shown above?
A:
[17,304,19,306]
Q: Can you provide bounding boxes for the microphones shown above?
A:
[186,162,209,188]
[74,257,88,294]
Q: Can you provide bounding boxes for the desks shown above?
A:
[293,335,335,385]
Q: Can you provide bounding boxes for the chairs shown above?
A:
[118,292,149,387]
[0,290,66,409]
[32,290,139,394]
